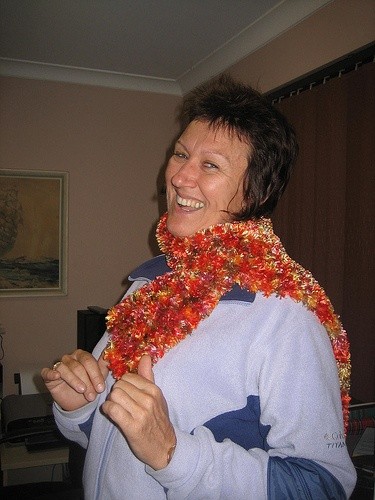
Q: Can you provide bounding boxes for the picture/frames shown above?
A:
[0,169,69,297]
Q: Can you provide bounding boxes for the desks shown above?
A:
[1,433,70,489]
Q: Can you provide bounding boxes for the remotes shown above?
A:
[87,305,107,315]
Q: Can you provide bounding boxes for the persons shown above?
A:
[40,75,358,500]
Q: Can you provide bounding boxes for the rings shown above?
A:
[53,361,63,371]
